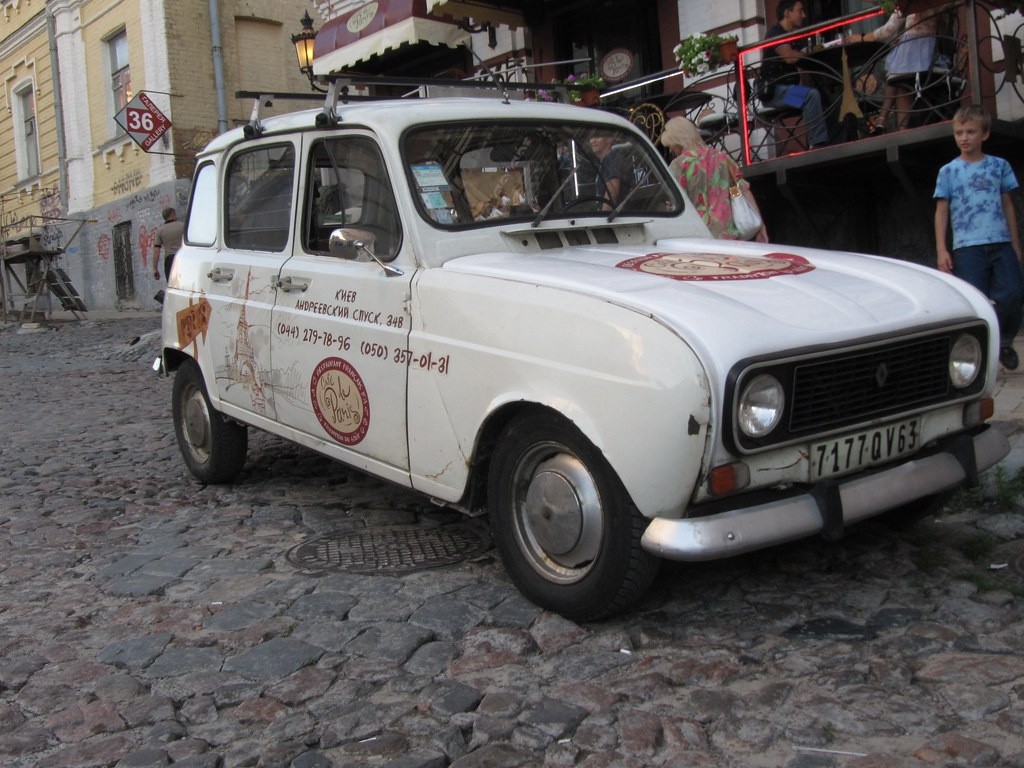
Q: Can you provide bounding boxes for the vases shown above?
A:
[713,39,739,68]
[571,87,601,107]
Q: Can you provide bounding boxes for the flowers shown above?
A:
[673,31,734,79]
[537,72,605,103]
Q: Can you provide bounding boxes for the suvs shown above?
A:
[151,71,1012,627]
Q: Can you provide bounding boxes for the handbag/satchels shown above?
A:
[727,184,762,240]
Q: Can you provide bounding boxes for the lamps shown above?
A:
[290,7,349,105]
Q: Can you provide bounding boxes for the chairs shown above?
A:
[664,67,809,166]
[852,11,969,135]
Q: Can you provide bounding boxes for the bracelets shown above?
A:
[861,32,866,42]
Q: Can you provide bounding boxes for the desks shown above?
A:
[782,41,892,147]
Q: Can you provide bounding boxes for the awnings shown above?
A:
[425,0,528,31]
[312,0,471,76]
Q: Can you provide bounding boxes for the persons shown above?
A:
[152,207,185,283]
[661,117,757,241]
[843,0,952,132]
[757,0,861,150]
[589,136,634,212]
[473,183,526,222]
[932,105,1024,373]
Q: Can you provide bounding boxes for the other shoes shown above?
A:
[811,142,831,149]
[998,346,1019,370]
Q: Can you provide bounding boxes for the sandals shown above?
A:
[869,116,887,136]
